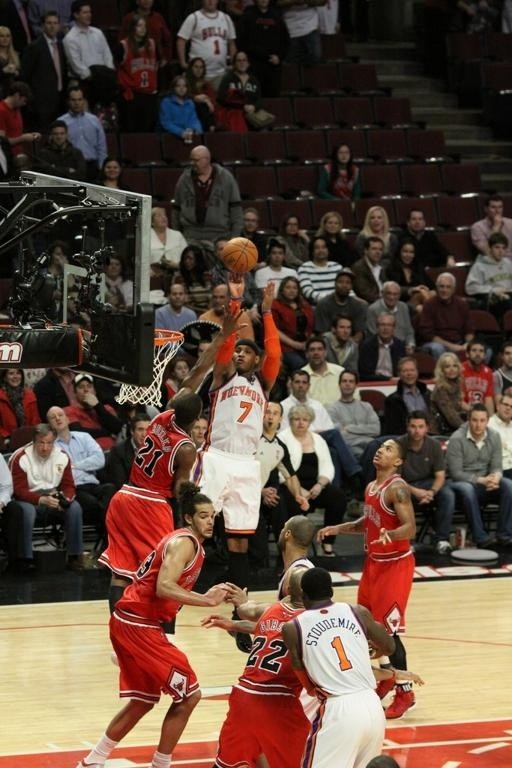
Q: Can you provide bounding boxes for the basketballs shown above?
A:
[223,237,258,273]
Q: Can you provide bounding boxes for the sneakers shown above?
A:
[385,687,414,718]
[375,671,395,700]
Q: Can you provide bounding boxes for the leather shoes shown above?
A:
[477,535,512,547]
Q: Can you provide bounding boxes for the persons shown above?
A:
[96,303,248,665]
[1,1,512,575]
[79,482,230,768]
[200,515,316,633]
[283,567,395,768]
[190,271,280,652]
[213,566,423,767]
[317,439,416,719]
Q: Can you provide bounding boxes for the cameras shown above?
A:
[52,491,69,509]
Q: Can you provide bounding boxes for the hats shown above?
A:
[72,374,94,385]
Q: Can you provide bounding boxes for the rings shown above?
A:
[231,280,233,281]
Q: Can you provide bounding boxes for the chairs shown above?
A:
[0,0,512,568]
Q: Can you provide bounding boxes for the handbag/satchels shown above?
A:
[246,110,274,129]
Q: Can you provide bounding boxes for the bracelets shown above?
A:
[231,296,241,300]
